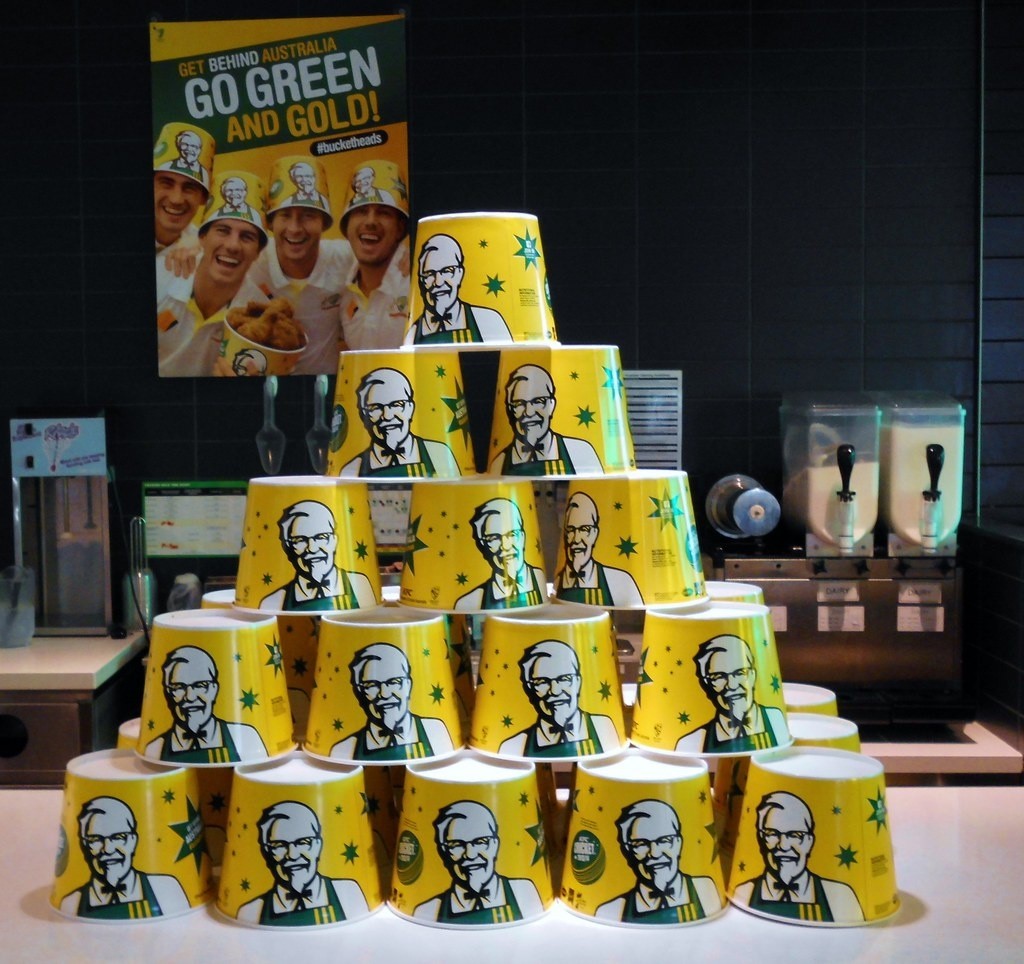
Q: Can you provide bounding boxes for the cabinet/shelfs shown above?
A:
[1,659,144,789]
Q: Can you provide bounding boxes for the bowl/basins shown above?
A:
[338,158,410,238]
[198,170,269,249]
[215,307,311,376]
[265,155,334,232]
[153,122,216,195]
[47,212,898,927]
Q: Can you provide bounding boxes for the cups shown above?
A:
[0,562,36,648]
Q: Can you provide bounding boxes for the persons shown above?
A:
[339,159,410,350]
[159,176,268,366]
[165,155,412,379]
[155,170,204,257]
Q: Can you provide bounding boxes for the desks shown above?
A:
[0,786,1024,964]
